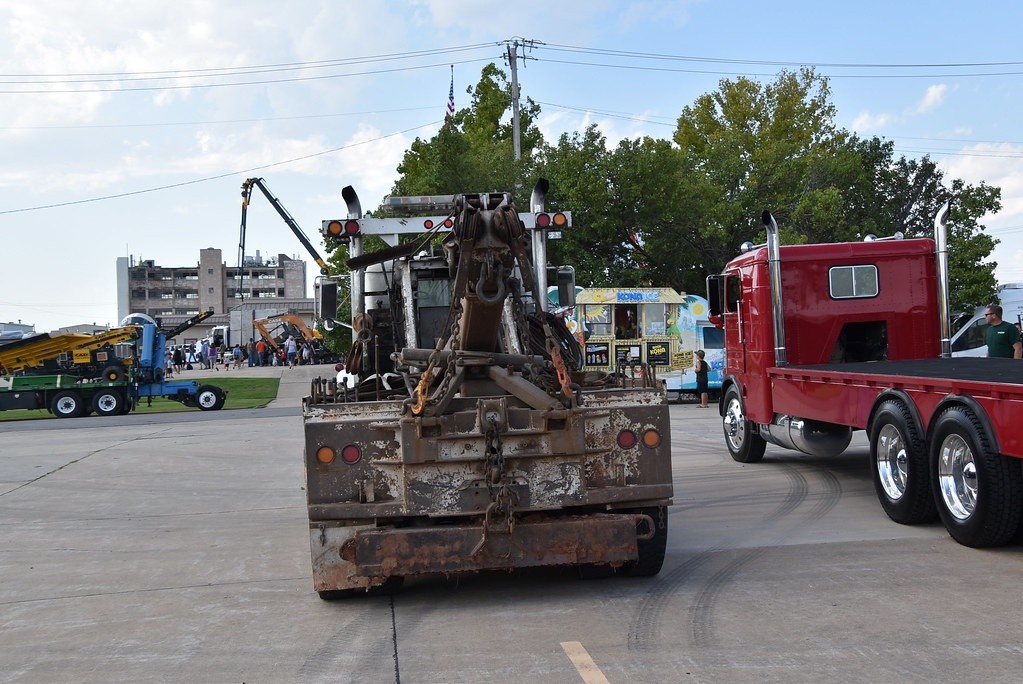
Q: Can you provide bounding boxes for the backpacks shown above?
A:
[234,352,239,360]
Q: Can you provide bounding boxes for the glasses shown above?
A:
[985,313,994,316]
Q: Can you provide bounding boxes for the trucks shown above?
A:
[950,282,1023,357]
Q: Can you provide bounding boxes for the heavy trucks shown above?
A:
[705,200,1023,549]
[0,310,226,418]
[195,325,248,361]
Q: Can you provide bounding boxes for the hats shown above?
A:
[694,350,705,358]
[335,364,344,371]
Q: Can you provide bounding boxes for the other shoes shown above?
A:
[233,367,234,369]
[215,367,219,371]
[696,404,709,408]
[249,364,255,367]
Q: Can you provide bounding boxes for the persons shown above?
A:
[694,350,711,408]
[983,305,1023,359]
[334,363,355,388]
[165,334,324,379]
[1014,322,1023,341]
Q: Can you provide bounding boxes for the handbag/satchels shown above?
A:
[187,364,193,370]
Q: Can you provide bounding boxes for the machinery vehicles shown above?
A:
[252,313,340,364]
[238,177,331,300]
[302,176,675,601]
